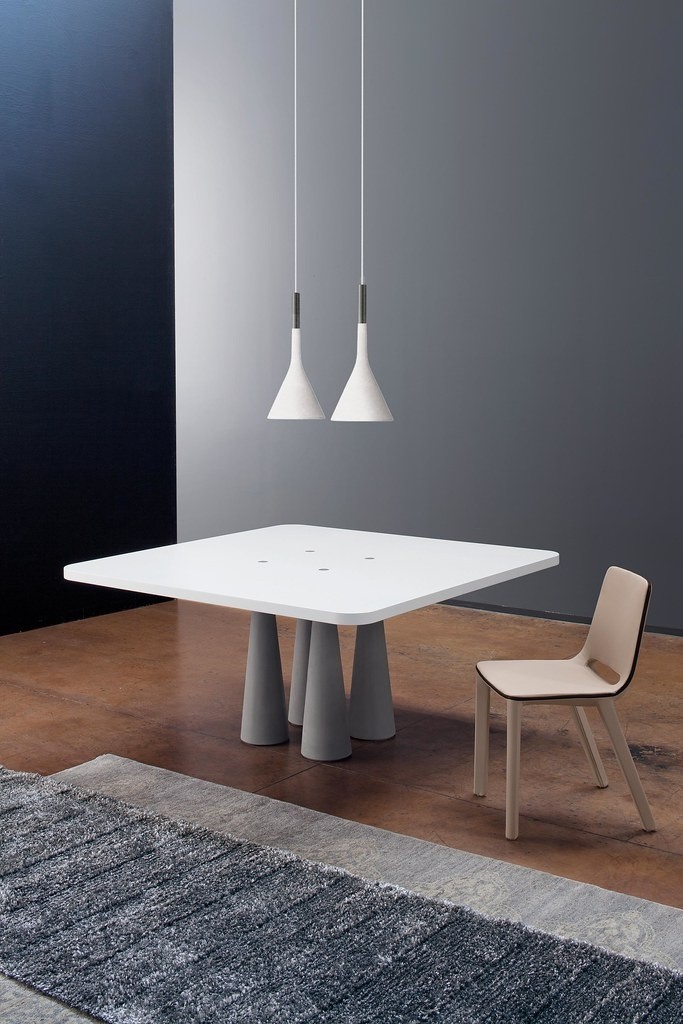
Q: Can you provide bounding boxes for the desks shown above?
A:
[64,525,560,759]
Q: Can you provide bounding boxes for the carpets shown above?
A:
[0,753,683,1024]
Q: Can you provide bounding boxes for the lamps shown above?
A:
[330,0,401,422]
[267,0,328,420]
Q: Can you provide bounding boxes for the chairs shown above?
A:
[473,565,656,838]
